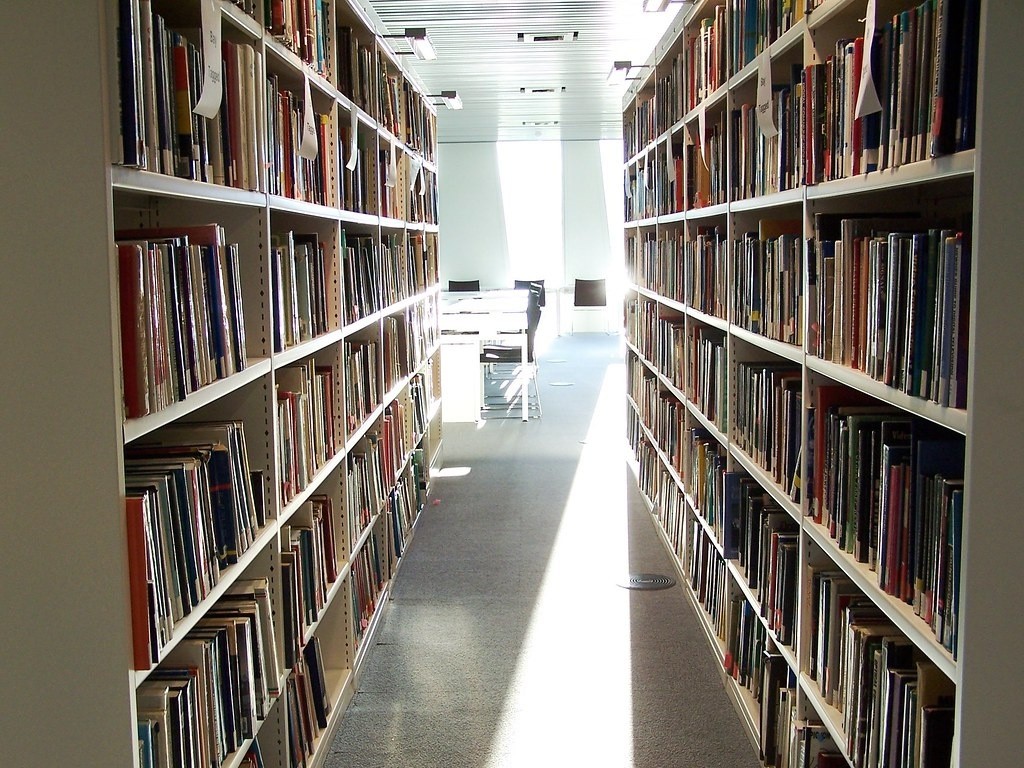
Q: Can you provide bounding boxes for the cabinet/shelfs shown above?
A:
[619,0,1024,768]
[0,0,442,768]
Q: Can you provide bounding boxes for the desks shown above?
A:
[441,289,529,422]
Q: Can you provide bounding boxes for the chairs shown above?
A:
[480,280,545,419]
[449,280,480,292]
[570,278,611,336]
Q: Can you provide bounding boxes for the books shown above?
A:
[113,0,441,768]
[622,0,981,768]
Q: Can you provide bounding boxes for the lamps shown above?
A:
[425,90,462,110]
[383,28,438,62]
[606,61,649,87]
[643,0,694,12]
